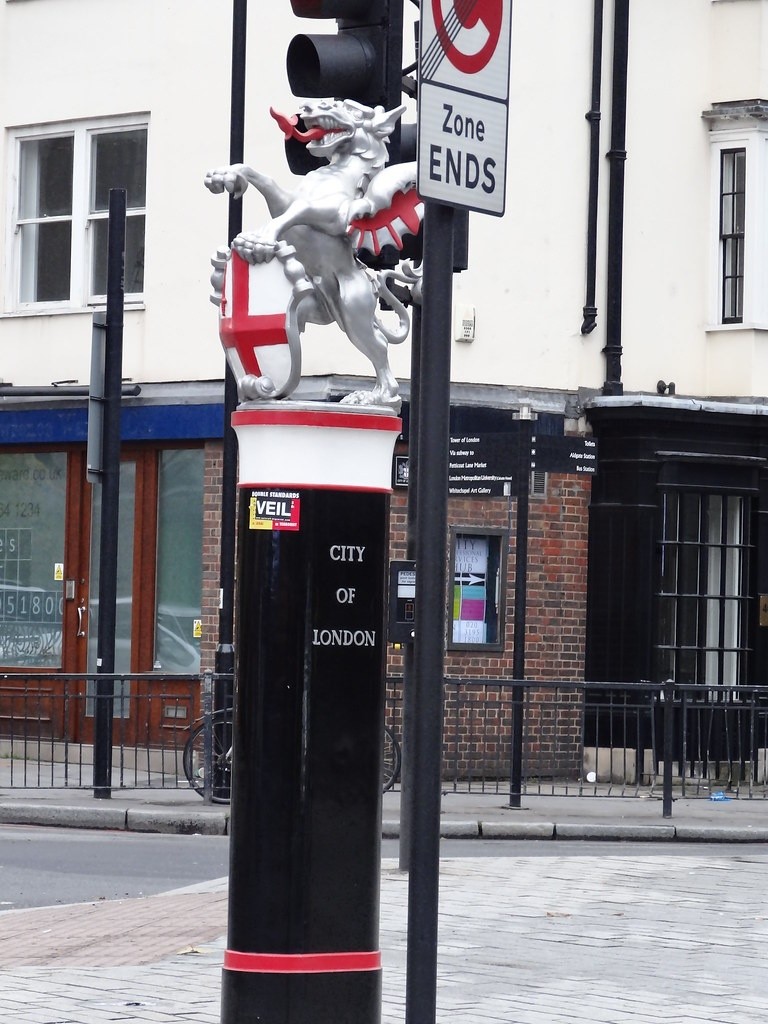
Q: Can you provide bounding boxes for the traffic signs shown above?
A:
[393,432,598,500]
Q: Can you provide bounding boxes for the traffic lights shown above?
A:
[290,0,402,174]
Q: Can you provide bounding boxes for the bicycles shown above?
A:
[180,706,402,803]
